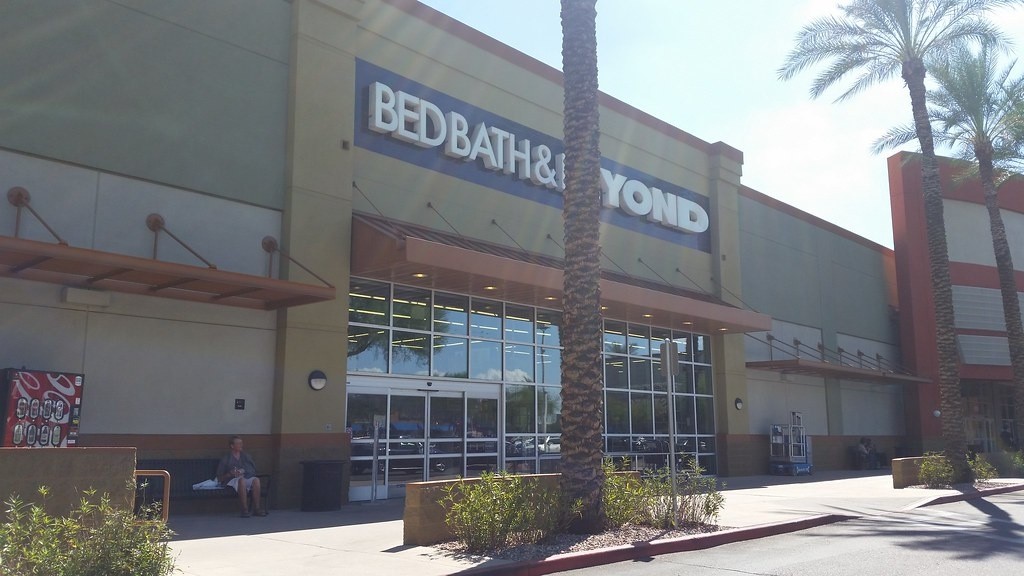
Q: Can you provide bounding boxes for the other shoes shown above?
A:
[252,509,266,517]
[241,510,250,518]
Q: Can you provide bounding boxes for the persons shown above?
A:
[216,436,267,518]
[1001,424,1013,452]
[859,438,881,470]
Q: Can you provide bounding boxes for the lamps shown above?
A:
[308,371,327,390]
[735,397,743,410]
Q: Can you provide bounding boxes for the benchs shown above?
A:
[849,445,889,469]
[894,446,921,457]
[135,457,272,518]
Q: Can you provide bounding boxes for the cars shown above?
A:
[602,434,707,464]
[351,432,560,473]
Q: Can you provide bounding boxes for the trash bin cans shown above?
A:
[299,460,344,512]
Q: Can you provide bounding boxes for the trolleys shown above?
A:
[768,411,817,477]
